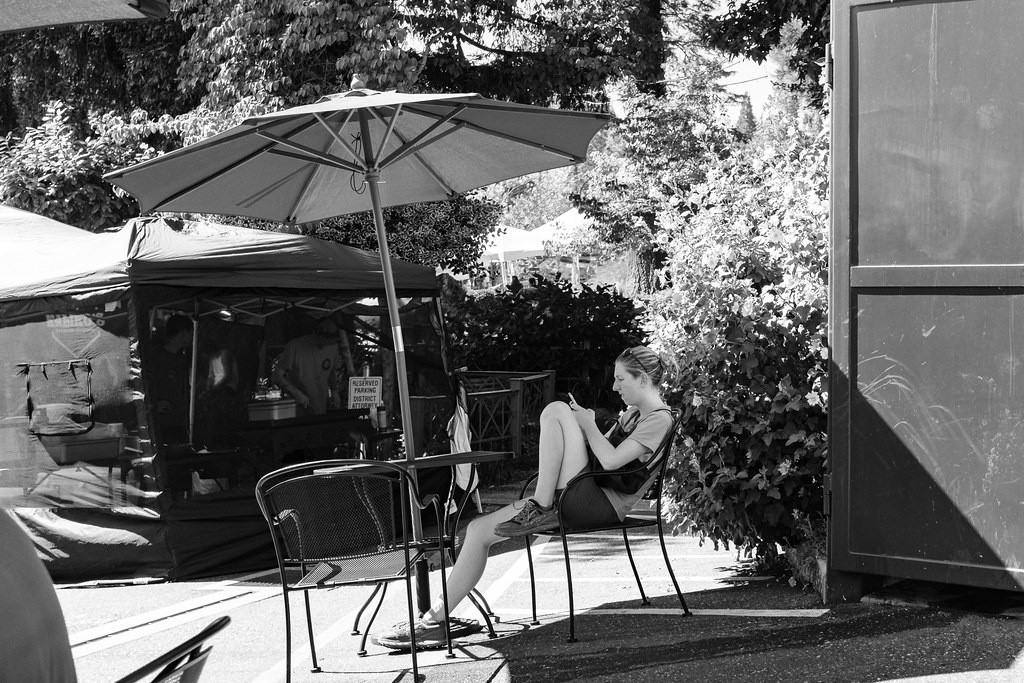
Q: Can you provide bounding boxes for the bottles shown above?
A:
[363,361,370,377]
[377,400,387,432]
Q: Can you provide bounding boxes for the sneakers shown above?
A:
[377,612,448,649]
[494,499,560,537]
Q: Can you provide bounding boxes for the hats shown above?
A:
[316,318,338,346]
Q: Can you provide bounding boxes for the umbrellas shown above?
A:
[102,75,618,544]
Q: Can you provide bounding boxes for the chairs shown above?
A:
[519,407,691,645]
[255,459,455,683]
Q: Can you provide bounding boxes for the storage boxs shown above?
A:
[248,398,299,421]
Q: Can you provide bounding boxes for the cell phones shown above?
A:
[568,392,578,405]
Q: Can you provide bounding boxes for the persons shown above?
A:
[268,316,342,416]
[379,344,681,651]
[198,337,242,418]
[145,312,193,445]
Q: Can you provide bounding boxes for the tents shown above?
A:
[0,212,485,583]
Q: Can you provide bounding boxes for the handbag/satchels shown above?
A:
[586,408,675,494]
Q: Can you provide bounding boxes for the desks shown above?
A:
[313,450,517,656]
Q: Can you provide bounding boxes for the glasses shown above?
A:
[622,347,647,373]
